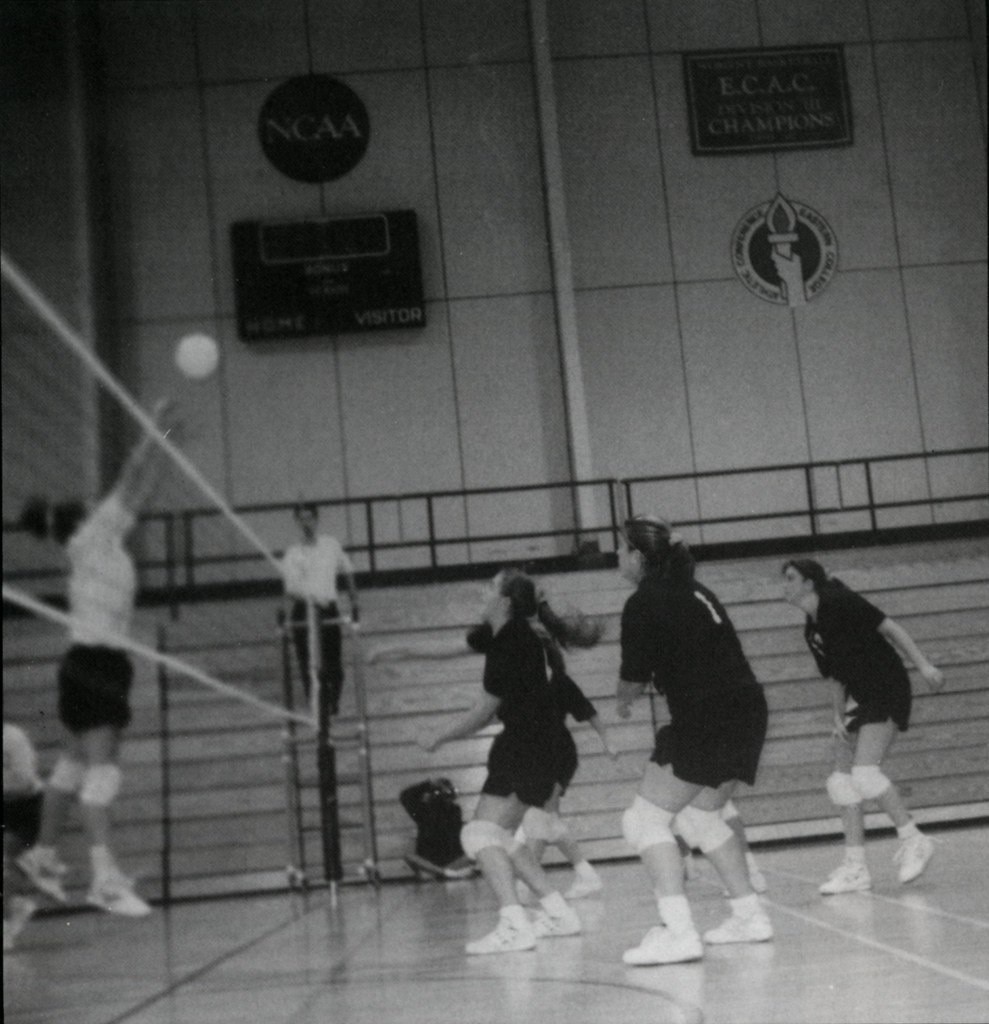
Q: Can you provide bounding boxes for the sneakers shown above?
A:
[819,857,871,893]
[531,907,582,938]
[622,917,704,964]
[685,859,699,879]
[14,844,68,903]
[86,875,152,918]
[724,873,767,896]
[3,895,36,949]
[564,874,603,899]
[892,830,935,882]
[464,917,537,953]
[703,907,773,944]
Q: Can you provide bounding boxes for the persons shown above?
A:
[782,559,947,894]
[275,503,361,726]
[16,395,187,918]
[614,515,772,965]
[415,568,619,955]
[3,721,44,951]
[400,777,477,879]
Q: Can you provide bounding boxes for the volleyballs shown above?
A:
[172,332,222,382]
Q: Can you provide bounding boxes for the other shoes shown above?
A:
[456,866,472,878]
